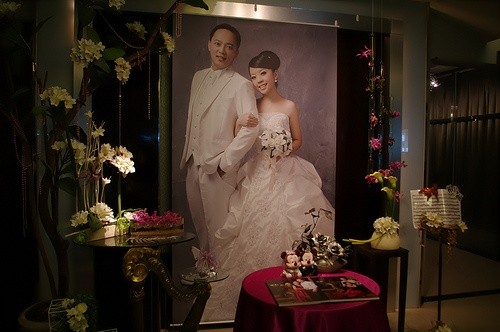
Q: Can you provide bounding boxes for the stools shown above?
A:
[353,242,410,332]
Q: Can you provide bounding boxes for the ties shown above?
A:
[207,72,217,87]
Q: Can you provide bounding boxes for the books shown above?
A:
[265,276,380,306]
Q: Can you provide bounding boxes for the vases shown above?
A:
[341,216,401,250]
[16,297,52,332]
[131,226,185,236]
[78,225,124,239]
[315,259,339,273]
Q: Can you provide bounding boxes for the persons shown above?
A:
[200,51,336,322]
[340,277,372,296]
[180,24,259,275]
[292,278,330,302]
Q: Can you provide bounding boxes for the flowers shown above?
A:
[371,170,400,233]
[417,184,467,332]
[258,128,292,160]
[355,46,411,183]
[0,1,186,332]
[311,232,350,264]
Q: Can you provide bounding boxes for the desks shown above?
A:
[71,231,228,332]
[232,268,391,331]
